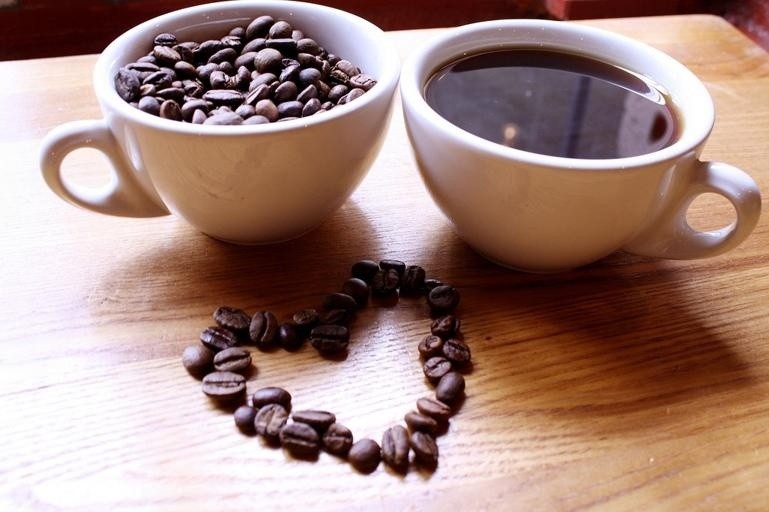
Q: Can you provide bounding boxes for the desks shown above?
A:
[0,14,768,511]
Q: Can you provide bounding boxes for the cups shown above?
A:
[39,0,400,246]
[399,19,761,274]
